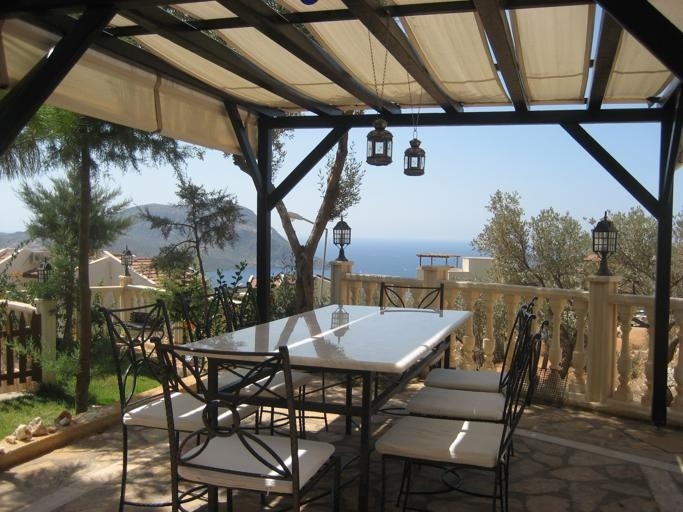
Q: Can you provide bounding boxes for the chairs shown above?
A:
[181,292,310,442]
[375,335,541,512]
[222,284,329,433]
[100,300,259,511]
[378,282,450,407]
[406,305,537,506]
[150,337,340,512]
[425,298,537,458]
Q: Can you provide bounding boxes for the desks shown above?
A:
[182,305,471,512]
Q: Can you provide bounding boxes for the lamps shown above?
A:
[404,71,428,176]
[334,217,352,262]
[366,10,392,165]
[592,212,618,276]
[121,247,132,277]
